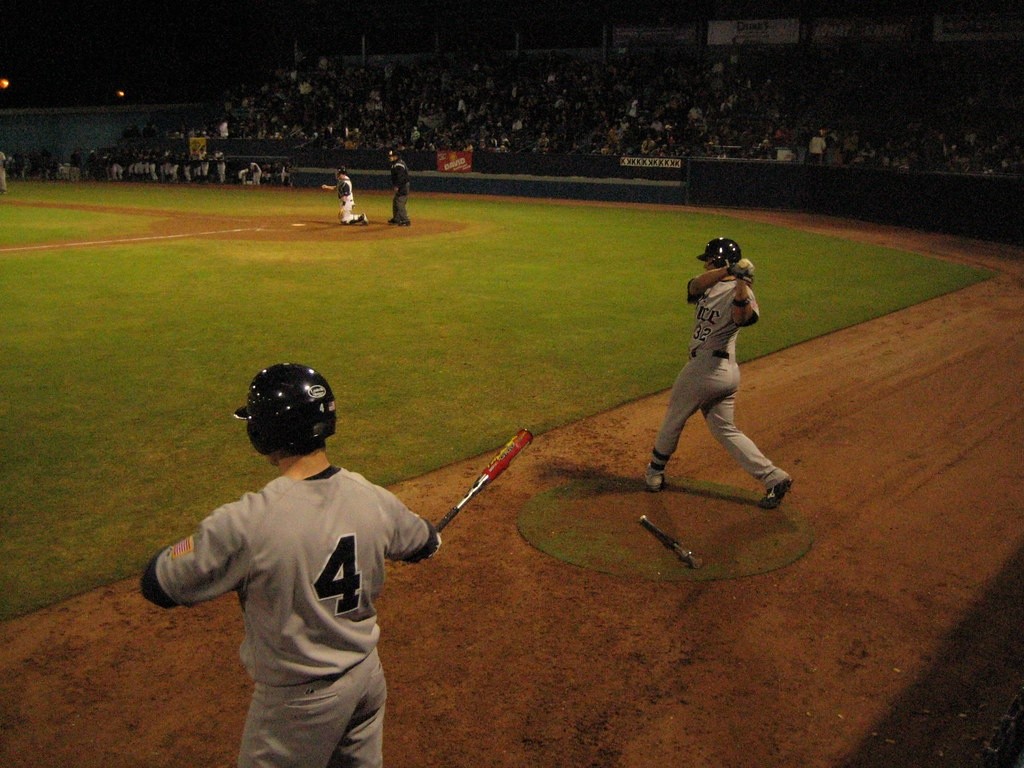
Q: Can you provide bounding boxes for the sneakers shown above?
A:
[760,478,794,508]
[644,462,664,492]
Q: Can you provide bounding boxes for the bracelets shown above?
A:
[733,299,750,307]
[733,290,751,297]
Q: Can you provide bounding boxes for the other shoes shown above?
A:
[398,220,411,225]
[362,213,368,224]
[388,219,398,224]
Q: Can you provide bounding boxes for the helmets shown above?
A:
[337,165,346,174]
[234,363,336,455]
[696,237,742,268]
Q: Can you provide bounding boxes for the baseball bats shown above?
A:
[639,513,703,569]
[433,427,534,529]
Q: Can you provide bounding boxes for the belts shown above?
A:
[691,350,729,359]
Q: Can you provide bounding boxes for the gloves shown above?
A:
[428,533,442,557]
[727,258,755,284]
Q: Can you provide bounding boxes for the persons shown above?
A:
[0,44,1024,194]
[387,150,410,225]
[140,364,442,768]
[321,167,369,225]
[644,237,794,508]
